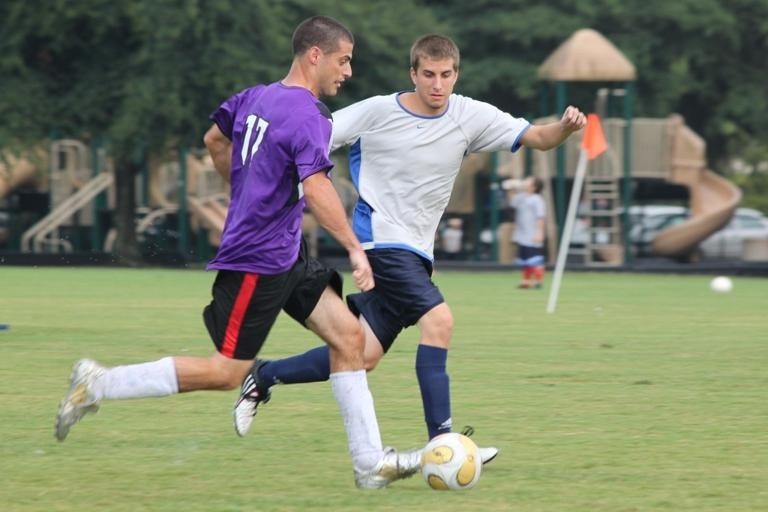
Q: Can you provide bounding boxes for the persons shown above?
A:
[52,11,425,493]
[438,216,463,259]
[230,29,589,473]
[568,213,594,250]
[507,171,550,292]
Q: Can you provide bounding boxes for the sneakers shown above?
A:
[425,441,499,472]
[353,445,437,491]
[54,357,107,443]
[232,356,273,437]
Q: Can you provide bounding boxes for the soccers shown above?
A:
[421,433,481,490]
[711,277,733,296]
